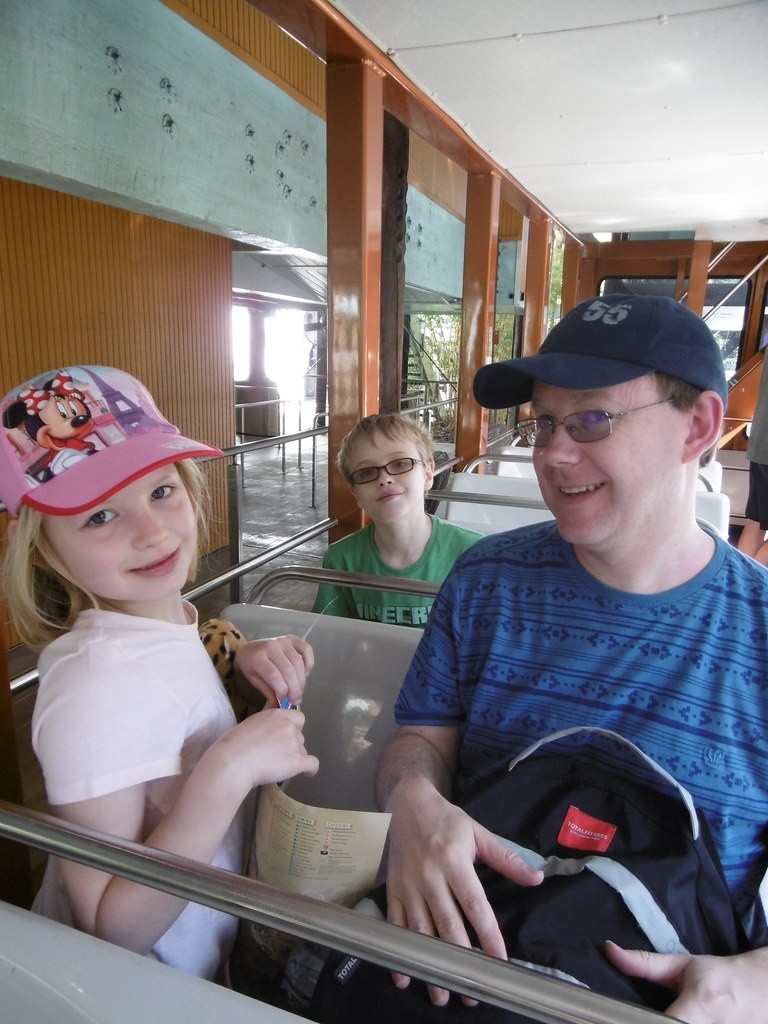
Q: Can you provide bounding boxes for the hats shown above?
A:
[0,363,224,517]
[473,293,729,417]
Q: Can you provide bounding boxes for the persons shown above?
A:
[373,288,768,1024]
[310,413,485,629]
[1,363,321,981]
[735,349,768,570]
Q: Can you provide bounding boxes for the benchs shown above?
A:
[0,802,684,1024]
[217,568,457,812]
[426,436,727,551]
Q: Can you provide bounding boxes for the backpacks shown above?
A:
[310,727,749,1024]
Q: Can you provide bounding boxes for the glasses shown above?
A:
[349,457,424,486]
[516,389,702,447]
